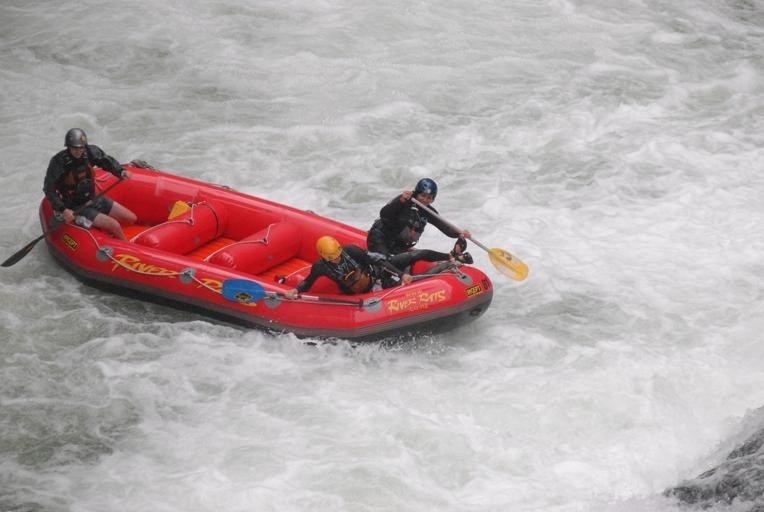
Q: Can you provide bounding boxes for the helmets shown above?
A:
[317,235,342,261]
[64,128,86,149]
[415,178,437,194]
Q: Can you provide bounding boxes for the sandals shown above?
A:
[450,237,473,264]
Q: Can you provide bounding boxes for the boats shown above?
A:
[37,162,496,346]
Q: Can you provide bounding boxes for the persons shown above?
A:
[42,128,138,242]
[284,178,473,301]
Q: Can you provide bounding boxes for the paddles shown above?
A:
[409,197,528,280]
[222,279,362,308]
[0,177,123,267]
